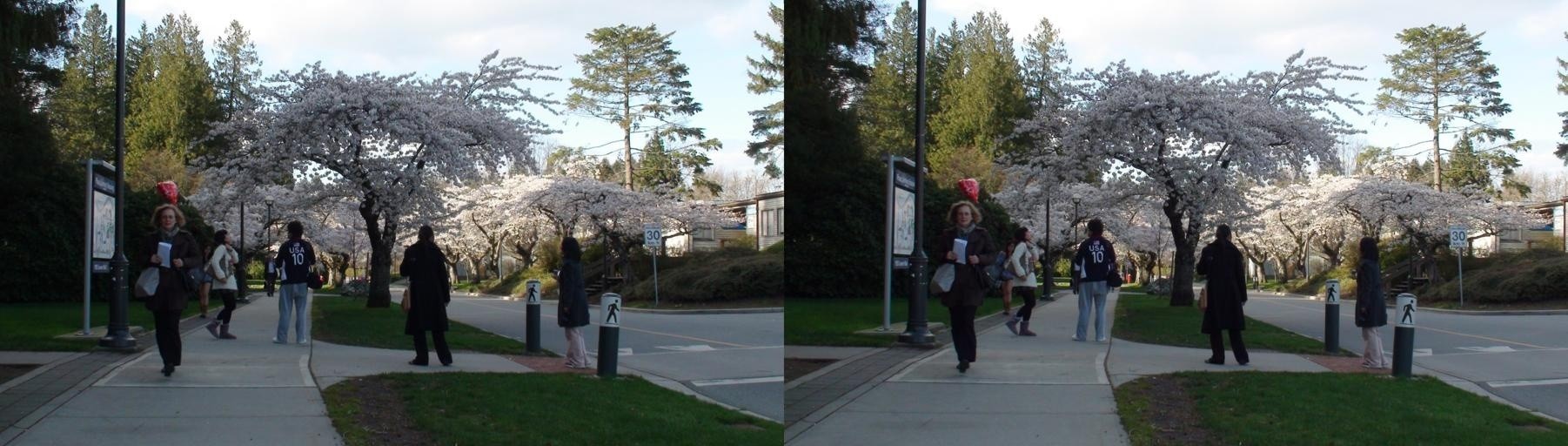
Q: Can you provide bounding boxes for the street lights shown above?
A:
[1070,193,1082,251]
[263,196,275,257]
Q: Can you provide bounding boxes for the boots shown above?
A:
[206,319,221,338]
[220,324,237,339]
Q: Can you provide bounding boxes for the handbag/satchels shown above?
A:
[203,255,236,283]
[132,267,161,299]
[307,271,321,289]
[179,267,205,296]
[401,289,411,311]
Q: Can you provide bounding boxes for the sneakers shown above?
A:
[564,362,573,368]
[299,339,307,344]
[272,337,279,343]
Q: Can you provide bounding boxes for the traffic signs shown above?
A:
[1451,225,1468,248]
[644,226,663,247]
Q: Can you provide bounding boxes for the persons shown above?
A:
[263,254,277,297]
[996,241,1016,315]
[1074,219,1116,344]
[273,221,317,344]
[1071,251,1080,293]
[1354,237,1388,369]
[936,201,996,372]
[1197,224,1248,366]
[131,205,202,376]
[551,237,592,368]
[199,245,212,317]
[1006,227,1045,336]
[206,229,239,342]
[400,226,453,366]
